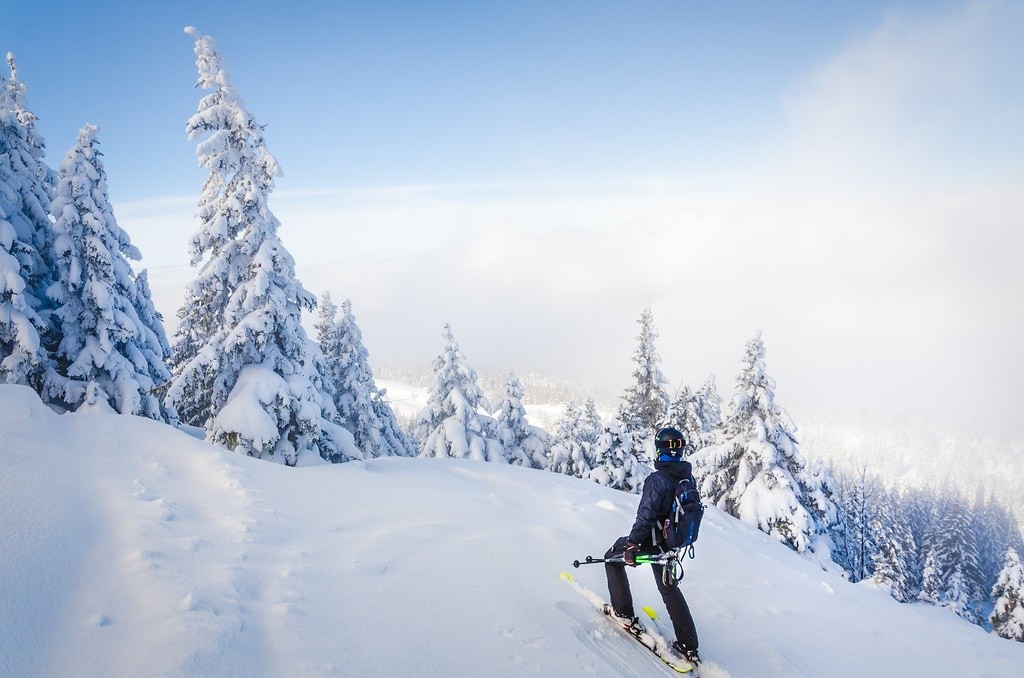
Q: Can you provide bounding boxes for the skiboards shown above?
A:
[559,571,706,678]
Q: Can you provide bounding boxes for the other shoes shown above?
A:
[604,604,635,628]
[671,640,699,661]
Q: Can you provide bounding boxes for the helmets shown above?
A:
[654,428,686,460]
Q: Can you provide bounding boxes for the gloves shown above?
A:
[623,542,640,567]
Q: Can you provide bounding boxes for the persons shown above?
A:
[603,428,701,664]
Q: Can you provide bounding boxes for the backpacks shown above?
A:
[651,471,705,551]
